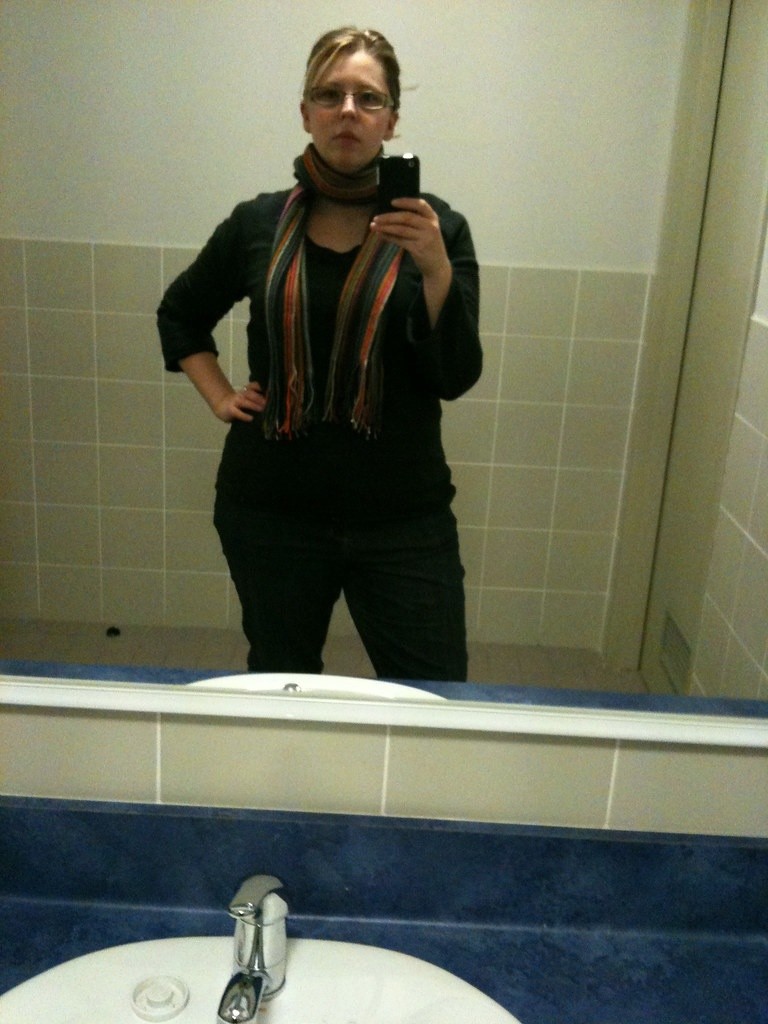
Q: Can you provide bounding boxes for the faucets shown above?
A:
[208,871,292,1024]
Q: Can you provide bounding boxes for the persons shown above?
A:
[153,25,487,687]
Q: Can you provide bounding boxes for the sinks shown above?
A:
[1,934,525,1024]
[178,670,451,707]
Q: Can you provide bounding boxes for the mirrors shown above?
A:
[0,0,768,718]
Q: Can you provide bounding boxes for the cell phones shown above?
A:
[375,154,421,216]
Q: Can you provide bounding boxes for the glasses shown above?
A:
[308,86,395,111]
